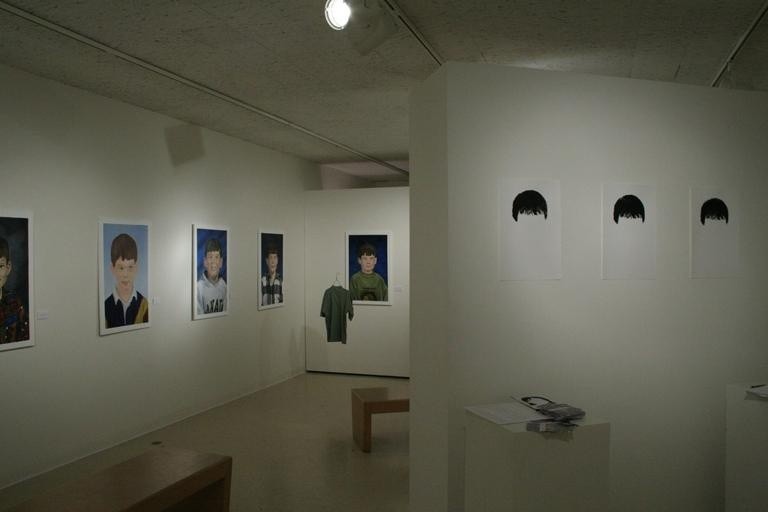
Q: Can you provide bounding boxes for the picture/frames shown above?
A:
[496,175,565,280]
[343,230,393,307]
[190,224,228,321]
[600,181,657,281]
[0,211,35,352]
[97,218,152,338]
[688,183,739,278]
[256,227,285,311]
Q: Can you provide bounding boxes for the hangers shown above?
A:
[330,272,344,287]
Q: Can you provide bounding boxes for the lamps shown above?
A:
[323,0,360,31]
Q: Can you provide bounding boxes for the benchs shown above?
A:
[1,443,244,509]
[350,385,409,453]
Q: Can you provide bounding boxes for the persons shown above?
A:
[350,242,387,301]
[0,238,29,343]
[197,238,227,314]
[262,239,283,308]
[103,233,148,329]
